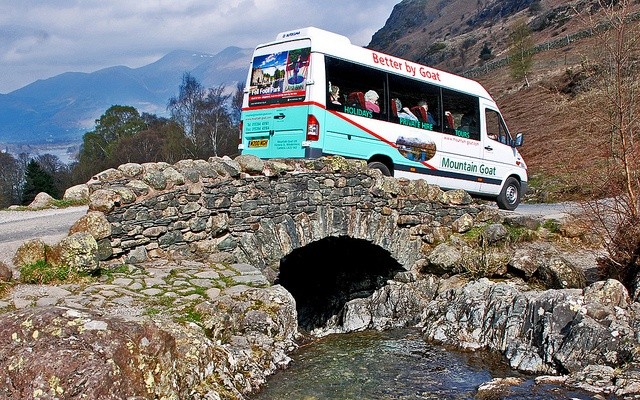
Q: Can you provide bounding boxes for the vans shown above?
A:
[238,26,528,210]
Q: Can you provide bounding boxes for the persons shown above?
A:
[329,86,342,105]
[363,90,381,114]
[417,100,437,125]
[288,55,309,84]
[394,97,419,122]
[444,110,452,129]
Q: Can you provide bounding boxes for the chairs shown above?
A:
[411,106,428,123]
[348,92,366,109]
[444,111,454,129]
[378,100,397,117]
[456,114,476,134]
